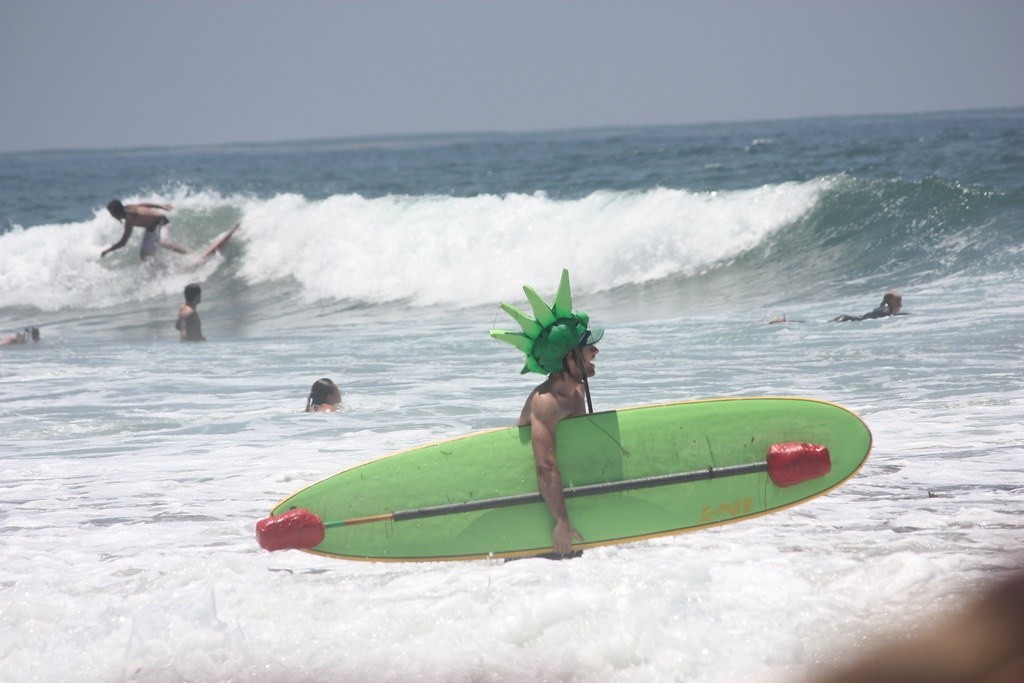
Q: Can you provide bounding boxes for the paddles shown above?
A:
[255,440,832,553]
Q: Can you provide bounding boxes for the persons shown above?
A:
[770,290,903,323]
[503,340,598,563]
[100,200,188,261]
[175,283,206,341]
[306,378,341,412]
[0,326,40,346]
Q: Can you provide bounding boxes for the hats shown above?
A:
[489,268,605,376]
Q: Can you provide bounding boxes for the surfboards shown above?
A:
[182,221,243,269]
[268,395,875,563]
